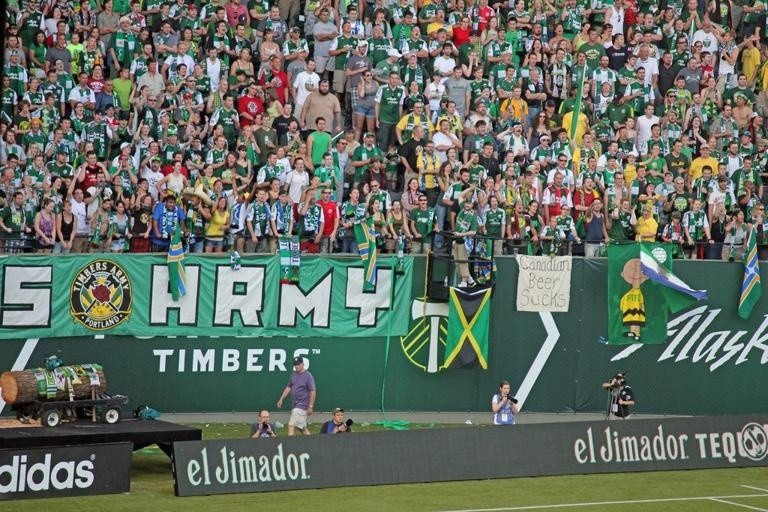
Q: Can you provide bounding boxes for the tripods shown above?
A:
[608,388,626,420]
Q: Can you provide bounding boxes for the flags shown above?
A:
[276,235,302,285]
[736,228,763,321]
[473,240,497,298]
[571,63,587,140]
[639,242,709,315]
[443,285,490,371]
[167,222,187,302]
[605,242,674,346]
[353,216,376,289]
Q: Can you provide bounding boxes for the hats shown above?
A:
[433,70,444,77]
[481,85,491,93]
[612,92,623,102]
[291,355,304,366]
[364,132,374,140]
[333,407,344,416]
[546,101,554,106]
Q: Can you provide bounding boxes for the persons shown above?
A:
[1,0,766,261]
[492,380,518,425]
[249,409,277,439]
[453,183,478,288]
[319,407,352,433]
[277,356,316,436]
[601,372,635,420]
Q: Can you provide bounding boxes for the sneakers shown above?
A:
[624,332,642,341]
[457,281,477,289]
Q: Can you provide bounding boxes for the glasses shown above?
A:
[322,192,331,194]
[559,159,567,162]
[370,185,378,187]
[365,74,372,77]
[539,116,545,118]
[541,139,549,142]
[339,142,348,145]
[668,95,676,98]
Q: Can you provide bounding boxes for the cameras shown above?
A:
[504,393,518,404]
[337,419,353,432]
[262,422,268,429]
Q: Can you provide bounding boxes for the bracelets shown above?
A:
[309,405,313,408]
